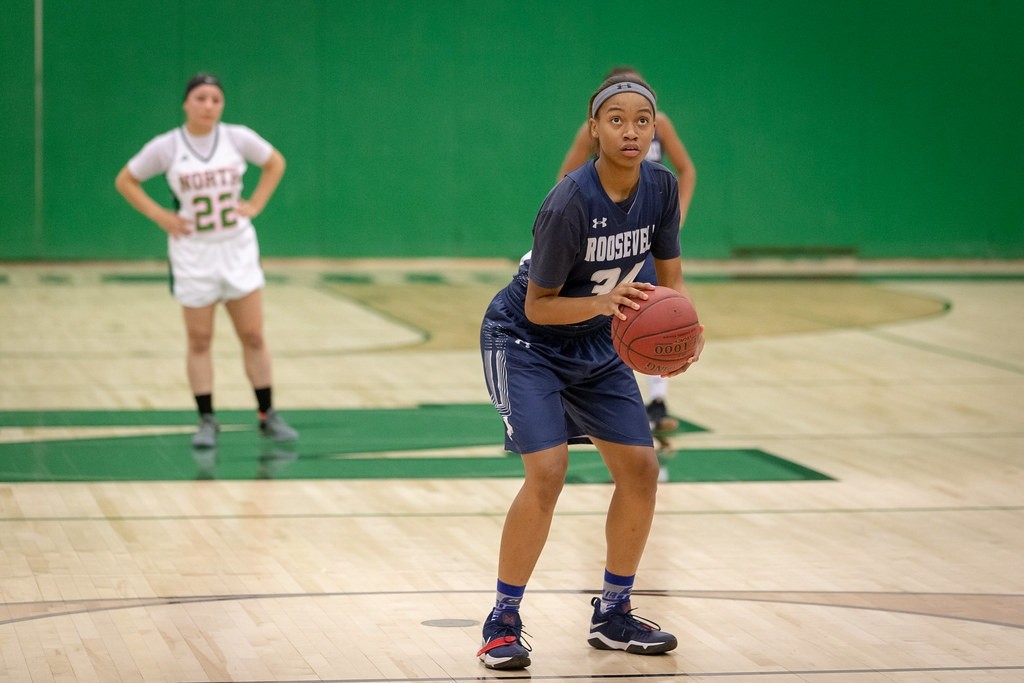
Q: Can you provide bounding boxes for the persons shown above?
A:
[115,75,298,448]
[475,74,706,672]
[560,68,696,430]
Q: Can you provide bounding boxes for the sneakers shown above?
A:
[258,406,297,441]
[476,607,532,669]
[192,413,219,448]
[587,596,678,654]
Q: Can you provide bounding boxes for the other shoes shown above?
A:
[647,399,677,430]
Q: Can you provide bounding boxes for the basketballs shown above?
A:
[611,285,700,375]
